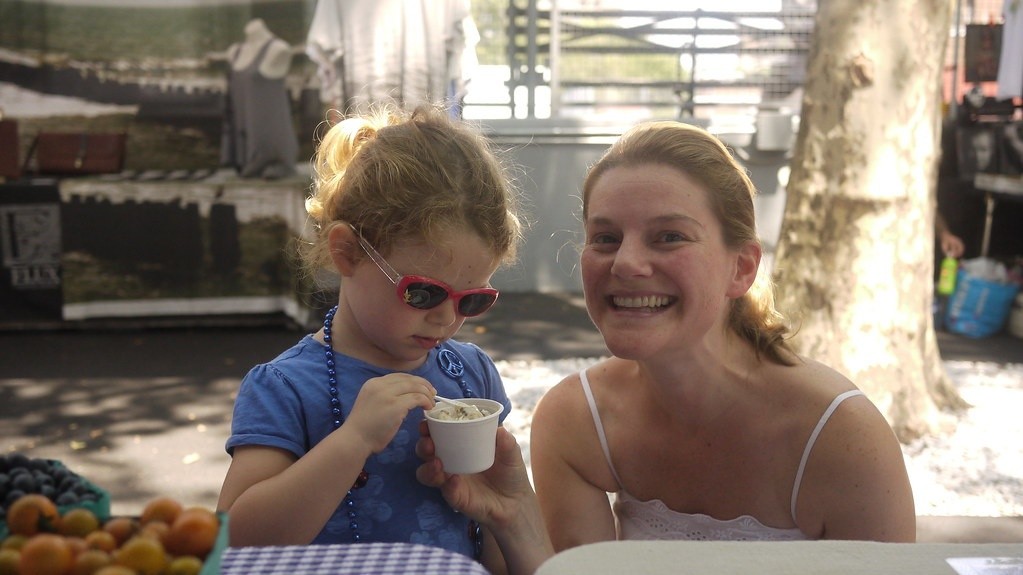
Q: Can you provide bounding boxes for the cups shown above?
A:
[423,396,503,475]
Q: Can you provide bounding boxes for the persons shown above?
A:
[934,205,964,260]
[966,131,993,172]
[225,17,297,180]
[217,98,535,574]
[417,122,915,574]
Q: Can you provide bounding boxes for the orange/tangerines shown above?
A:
[0,494,218,574]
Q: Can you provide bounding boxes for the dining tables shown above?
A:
[216,538,501,575]
[534,537,1022,575]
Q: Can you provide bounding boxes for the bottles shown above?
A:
[937,249,959,295]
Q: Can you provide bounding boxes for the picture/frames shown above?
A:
[956,124,1006,181]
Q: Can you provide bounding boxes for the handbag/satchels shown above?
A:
[944,260,1018,339]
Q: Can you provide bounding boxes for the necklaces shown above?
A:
[323,304,482,562]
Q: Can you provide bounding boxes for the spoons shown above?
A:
[434,394,481,417]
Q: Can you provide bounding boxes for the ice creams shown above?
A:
[428,405,494,421]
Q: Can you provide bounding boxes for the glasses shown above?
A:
[349,223,499,318]
